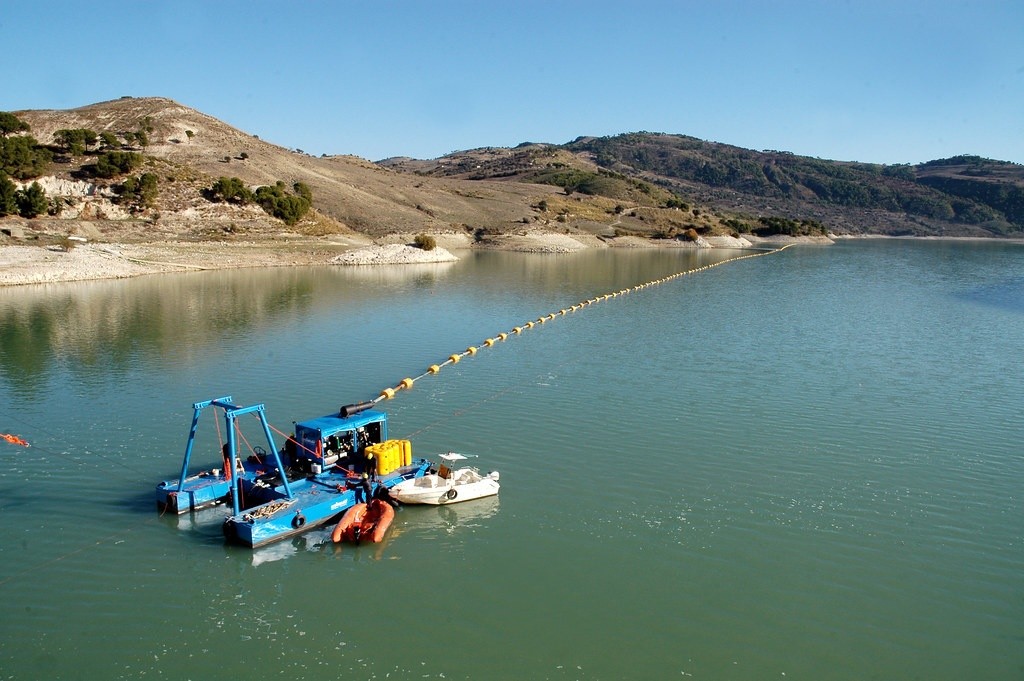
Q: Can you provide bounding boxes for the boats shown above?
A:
[154,393,435,549]
[387,452,501,506]
[331,499,395,544]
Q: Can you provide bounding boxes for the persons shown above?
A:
[221,441,230,473]
[345,442,355,456]
[353,473,373,510]
[364,453,376,482]
[285,433,298,466]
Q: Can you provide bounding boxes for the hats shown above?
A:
[363,473,368,479]
